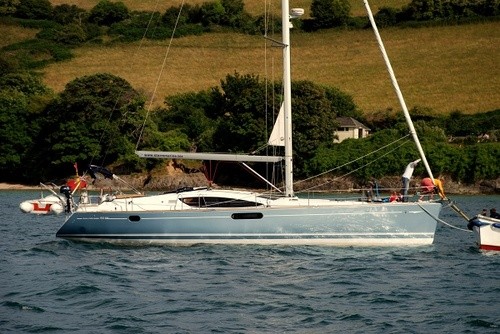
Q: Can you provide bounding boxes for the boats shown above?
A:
[467,215,500,253]
[19,194,67,214]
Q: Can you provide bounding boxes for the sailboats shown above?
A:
[56,0,442,245]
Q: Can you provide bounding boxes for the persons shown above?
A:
[417,176,448,202]
[401,159,422,202]
